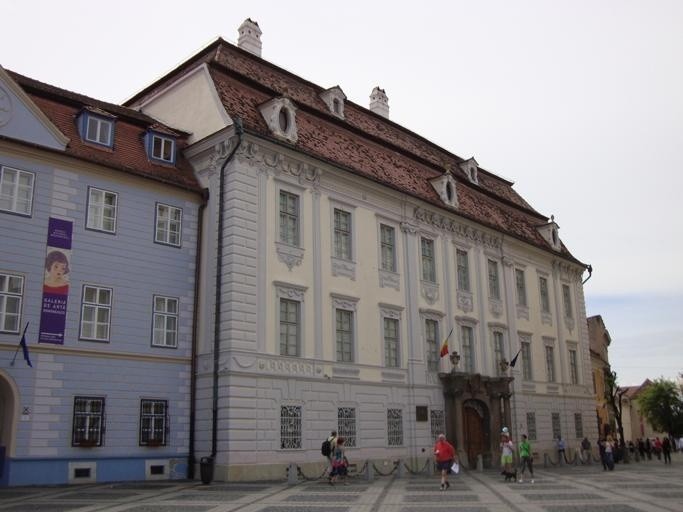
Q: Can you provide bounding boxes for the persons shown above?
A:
[580,436,590,461]
[432,433,459,492]
[596,432,670,472]
[42,250,68,296]
[325,429,339,481]
[327,436,350,487]
[499,430,516,479]
[518,433,535,485]
[554,434,566,464]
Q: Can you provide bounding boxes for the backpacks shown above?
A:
[321,437,335,456]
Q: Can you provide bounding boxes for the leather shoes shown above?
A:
[440,484,444,490]
[444,482,449,491]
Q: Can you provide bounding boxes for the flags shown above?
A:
[18,320,33,369]
[439,326,453,357]
[508,348,521,369]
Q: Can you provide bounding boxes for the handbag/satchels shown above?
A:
[337,464,347,476]
[451,462,460,474]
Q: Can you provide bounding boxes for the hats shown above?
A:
[502,427,509,432]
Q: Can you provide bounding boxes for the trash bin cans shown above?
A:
[200,457,213,484]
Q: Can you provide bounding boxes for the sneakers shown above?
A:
[322,477,350,486]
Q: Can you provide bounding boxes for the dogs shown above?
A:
[501,468,518,481]
